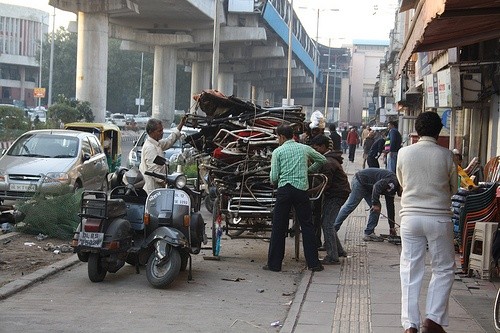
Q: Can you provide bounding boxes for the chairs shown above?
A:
[451,154,500,273]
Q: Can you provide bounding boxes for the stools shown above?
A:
[468,222,499,280]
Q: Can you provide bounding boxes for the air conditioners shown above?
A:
[461,72,482,102]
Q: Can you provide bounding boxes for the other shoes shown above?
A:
[319,258,341,265]
[264,265,280,271]
[405,328,417,333]
[362,233,384,242]
[421,318,446,333]
[310,265,324,272]
[319,244,326,252]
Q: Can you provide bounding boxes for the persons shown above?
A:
[262,128,325,272]
[394,111,459,333]
[138,114,187,196]
[333,165,404,243]
[102,135,111,155]
[311,135,352,265]
[312,120,401,174]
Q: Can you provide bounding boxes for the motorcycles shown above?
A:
[64,122,121,172]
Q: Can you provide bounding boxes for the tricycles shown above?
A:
[210,195,302,265]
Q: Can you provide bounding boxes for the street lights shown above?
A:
[297,5,340,115]
[315,36,344,116]
[38,14,56,109]
[323,53,349,120]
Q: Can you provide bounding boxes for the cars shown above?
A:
[127,129,196,170]
[104,109,152,131]
[0,100,51,127]
[0,130,108,223]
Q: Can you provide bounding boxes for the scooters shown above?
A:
[142,156,208,290]
[68,155,148,284]
